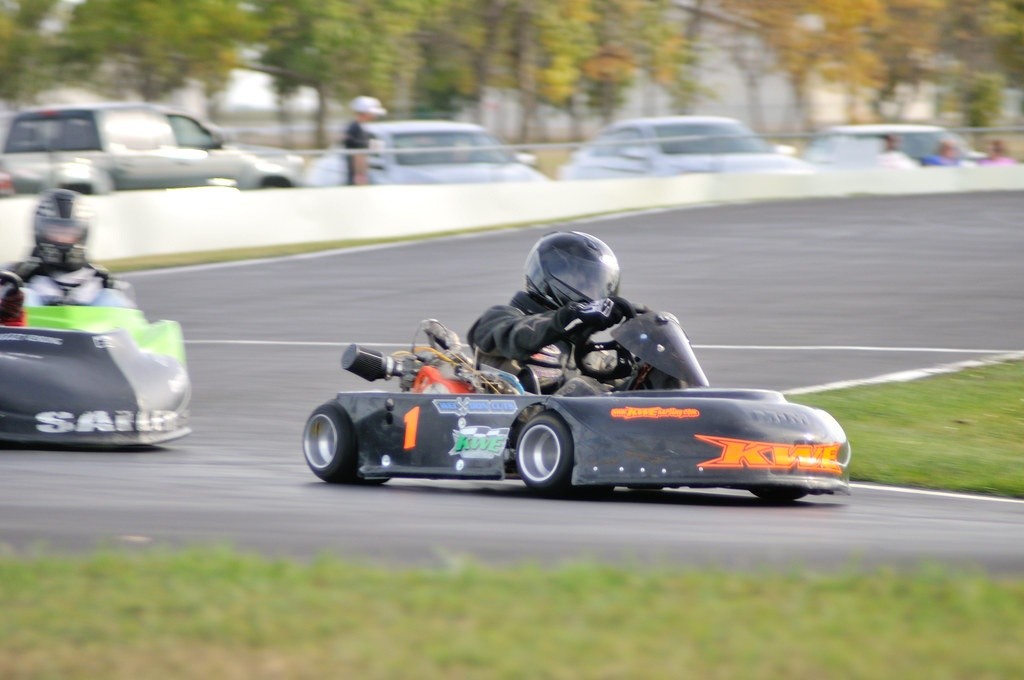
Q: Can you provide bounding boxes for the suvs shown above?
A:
[0,103,304,212]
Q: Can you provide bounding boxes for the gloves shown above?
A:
[3,285,24,316]
[558,295,646,329]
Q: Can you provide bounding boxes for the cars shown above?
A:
[561,115,826,190]
[313,118,558,190]
[801,125,1001,180]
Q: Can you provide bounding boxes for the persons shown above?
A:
[462,229,684,397]
[1,185,138,338]
[341,95,387,185]
[974,139,1020,165]
[872,133,921,168]
[922,140,968,167]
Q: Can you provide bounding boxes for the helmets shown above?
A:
[526,232,620,312]
[31,188,95,268]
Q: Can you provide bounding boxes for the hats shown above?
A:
[352,96,389,117]
